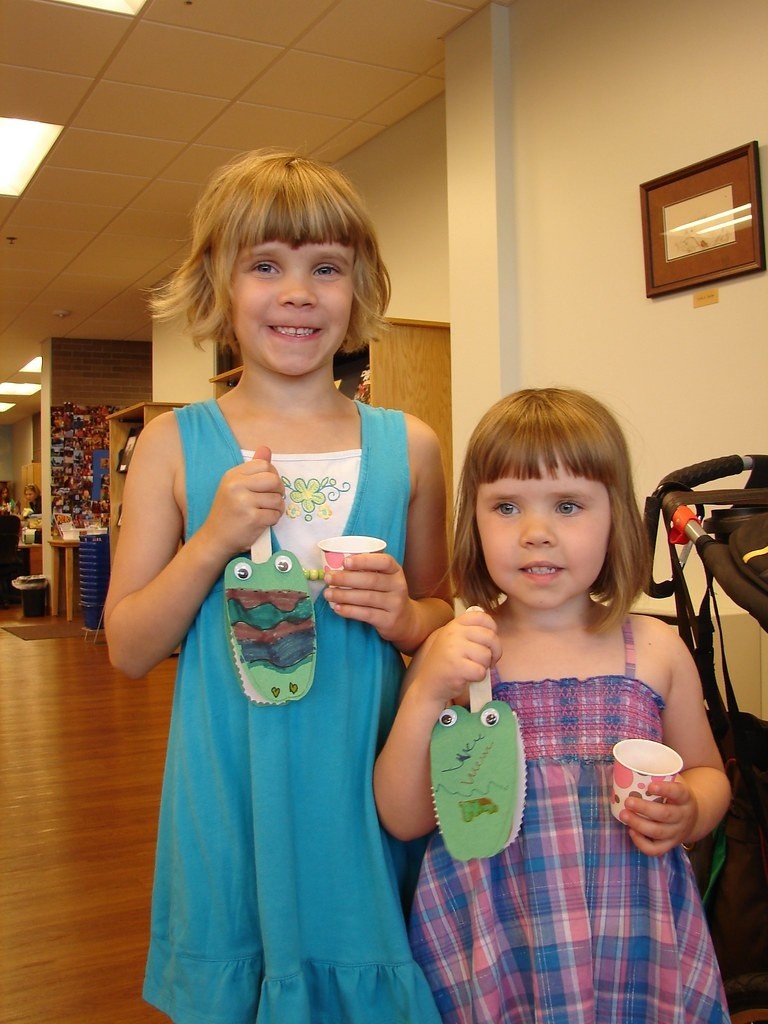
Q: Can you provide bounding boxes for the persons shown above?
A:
[24,482,41,514]
[0,482,19,513]
[372,385,736,1024]
[102,149,456,1023]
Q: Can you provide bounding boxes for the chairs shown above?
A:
[0,514,22,607]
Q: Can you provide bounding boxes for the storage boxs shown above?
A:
[78,533,111,631]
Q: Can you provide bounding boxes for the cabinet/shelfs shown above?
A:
[210,316,454,670]
[105,401,192,570]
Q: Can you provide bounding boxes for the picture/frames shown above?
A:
[637,141,766,299]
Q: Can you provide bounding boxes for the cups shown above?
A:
[611,738,683,825]
[317,534,388,610]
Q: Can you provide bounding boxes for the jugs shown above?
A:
[22,528,36,544]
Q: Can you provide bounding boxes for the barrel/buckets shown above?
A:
[19,575,45,617]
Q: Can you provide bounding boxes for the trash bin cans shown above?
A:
[11,575,49,618]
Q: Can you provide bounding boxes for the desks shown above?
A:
[17,538,42,574]
[47,539,81,622]
